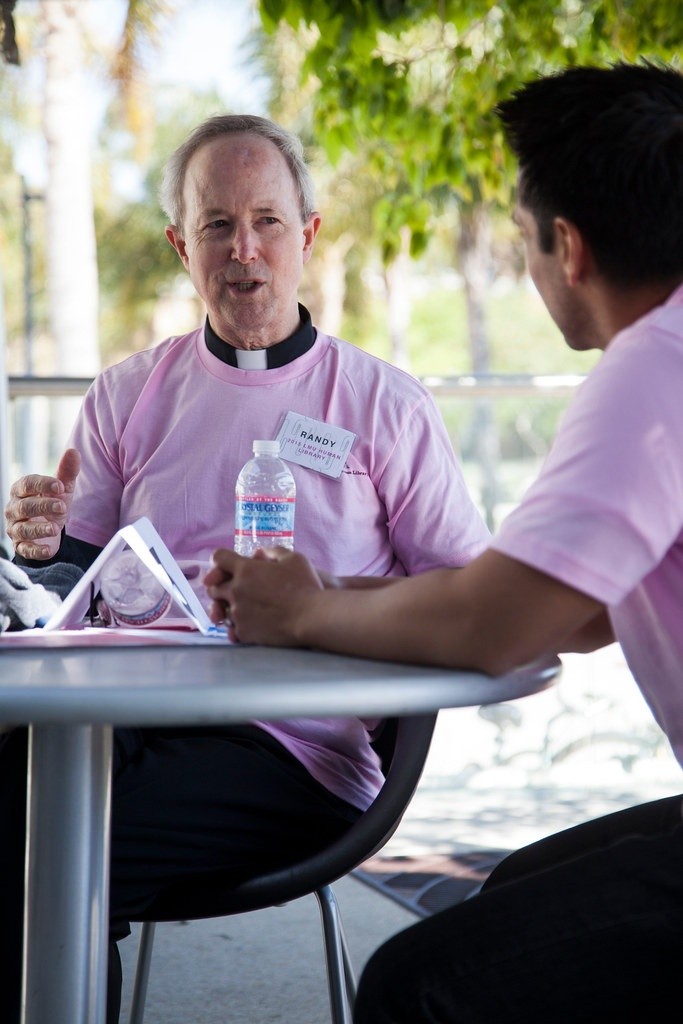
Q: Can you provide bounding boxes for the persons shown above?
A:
[2,116,493,1024]
[203,60,683,1024]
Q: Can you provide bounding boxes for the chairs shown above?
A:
[123,711,437,1024]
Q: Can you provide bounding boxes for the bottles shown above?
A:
[101,549,173,628]
[234,439,296,560]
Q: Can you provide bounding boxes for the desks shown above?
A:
[0,643,564,1024]
